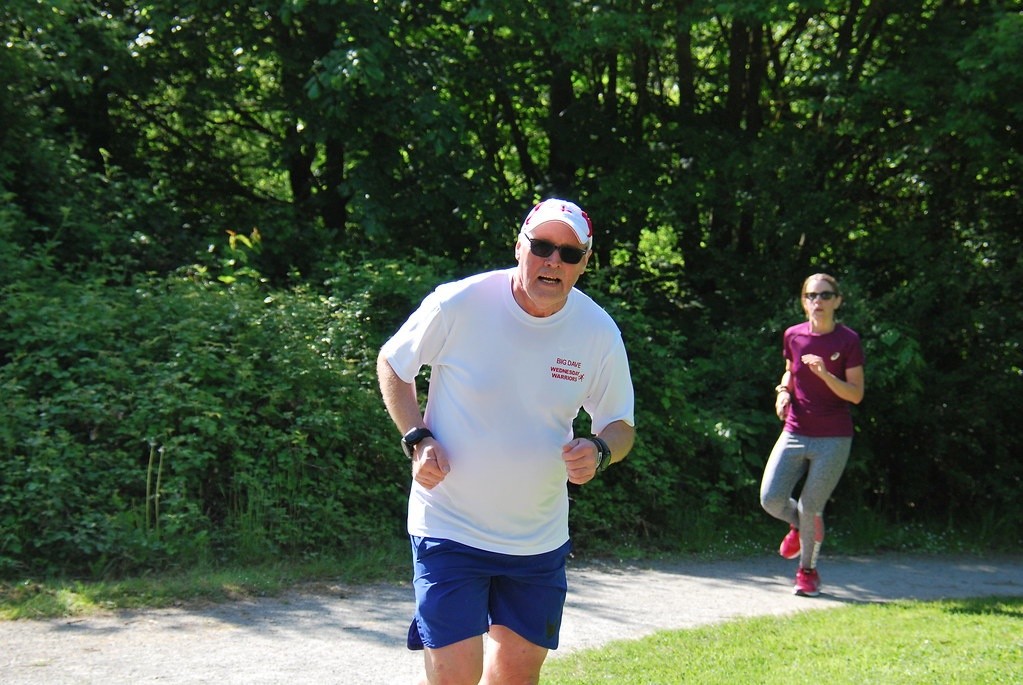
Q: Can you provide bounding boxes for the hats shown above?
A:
[521,198,594,250]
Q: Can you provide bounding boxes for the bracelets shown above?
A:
[775,386,788,392]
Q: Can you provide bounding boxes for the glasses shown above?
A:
[805,291,836,300]
[525,235,589,264]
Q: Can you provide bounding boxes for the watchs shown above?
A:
[401,428,434,459]
[587,432,612,473]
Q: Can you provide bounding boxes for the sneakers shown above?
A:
[794,567,821,597]
[779,523,801,559]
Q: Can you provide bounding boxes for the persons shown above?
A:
[760,273,864,596]
[378,196,636,685]
